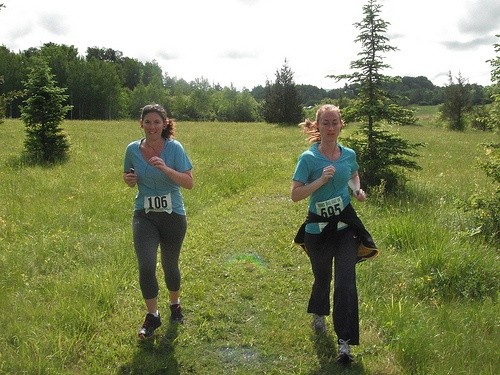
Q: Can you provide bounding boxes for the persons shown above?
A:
[122,103,194,340]
[290,104,379,363]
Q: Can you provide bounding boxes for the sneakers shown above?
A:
[314,315,325,333]
[170,304,182,322]
[337,339,352,362]
[139,313,161,338]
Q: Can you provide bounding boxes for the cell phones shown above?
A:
[129,168,134,175]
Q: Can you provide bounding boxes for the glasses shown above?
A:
[144,104,164,113]
